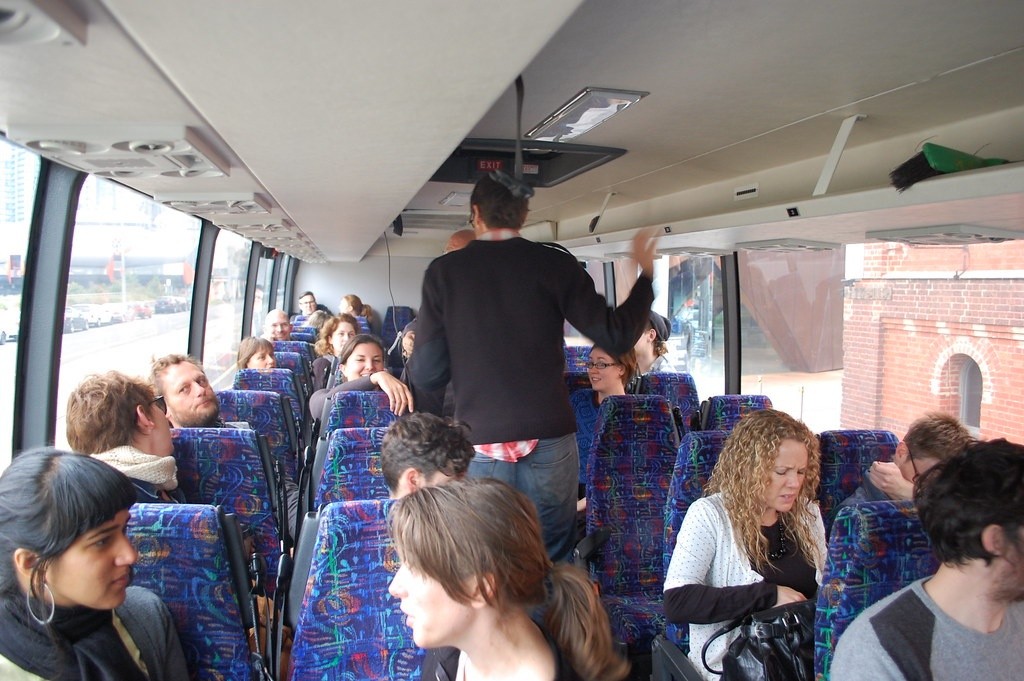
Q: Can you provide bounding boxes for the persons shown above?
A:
[312,313,360,391]
[65,370,186,507]
[568,341,636,542]
[236,336,277,370]
[662,408,828,681]
[387,476,632,681]
[263,309,293,341]
[404,171,665,564]
[830,438,1024,681]
[303,310,331,329]
[145,354,238,429]
[401,229,477,359]
[832,413,973,544]
[309,333,414,423]
[0,446,188,681]
[634,310,678,378]
[340,294,373,324]
[380,412,477,499]
[298,291,317,316]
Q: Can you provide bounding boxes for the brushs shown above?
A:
[887,134,1009,194]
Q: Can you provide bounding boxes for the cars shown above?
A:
[0,296,192,345]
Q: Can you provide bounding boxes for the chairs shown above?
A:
[123,305,937,681]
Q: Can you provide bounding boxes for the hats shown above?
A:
[650,311,671,341]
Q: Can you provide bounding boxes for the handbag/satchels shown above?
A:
[247,595,292,681]
[701,598,816,681]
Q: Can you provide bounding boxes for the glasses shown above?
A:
[467,210,476,229]
[145,395,167,416]
[586,362,618,369]
[907,444,921,482]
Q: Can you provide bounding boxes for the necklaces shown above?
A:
[764,514,786,560]
